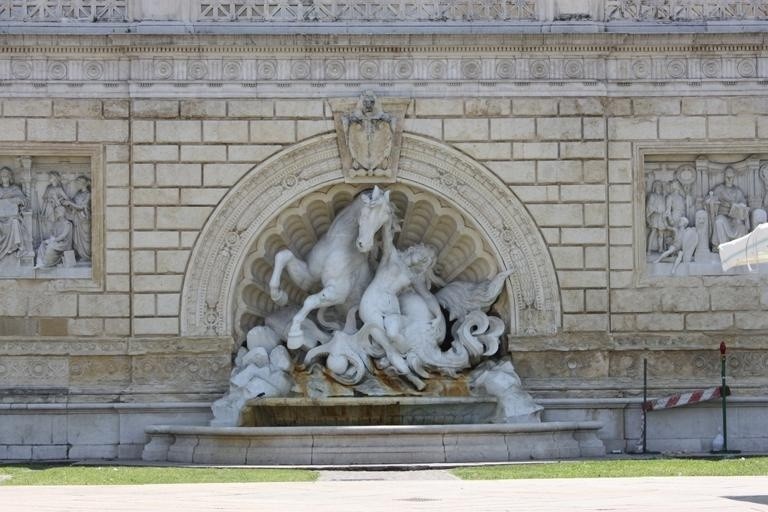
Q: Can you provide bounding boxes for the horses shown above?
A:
[268,184,519,369]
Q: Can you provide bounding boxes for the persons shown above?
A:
[0,167,90,271]
[358,219,446,390]
[647,168,750,273]
